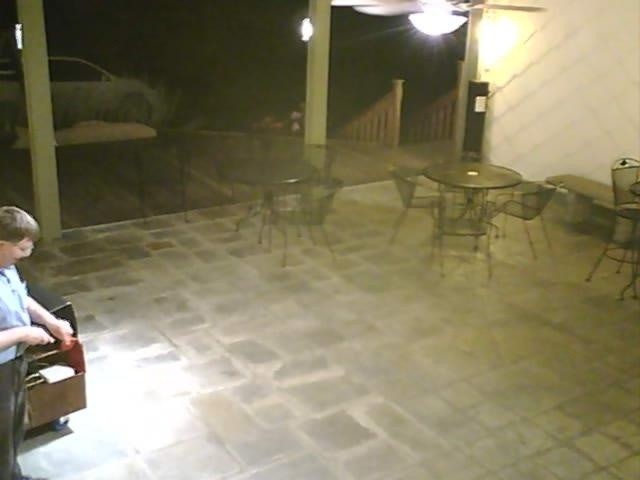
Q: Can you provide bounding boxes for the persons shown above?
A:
[0,205,74,480]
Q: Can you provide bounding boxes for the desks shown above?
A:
[223,151,308,244]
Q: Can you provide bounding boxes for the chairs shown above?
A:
[384,153,640,303]
[270,140,345,261]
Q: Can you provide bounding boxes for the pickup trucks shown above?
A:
[1,56,166,140]
